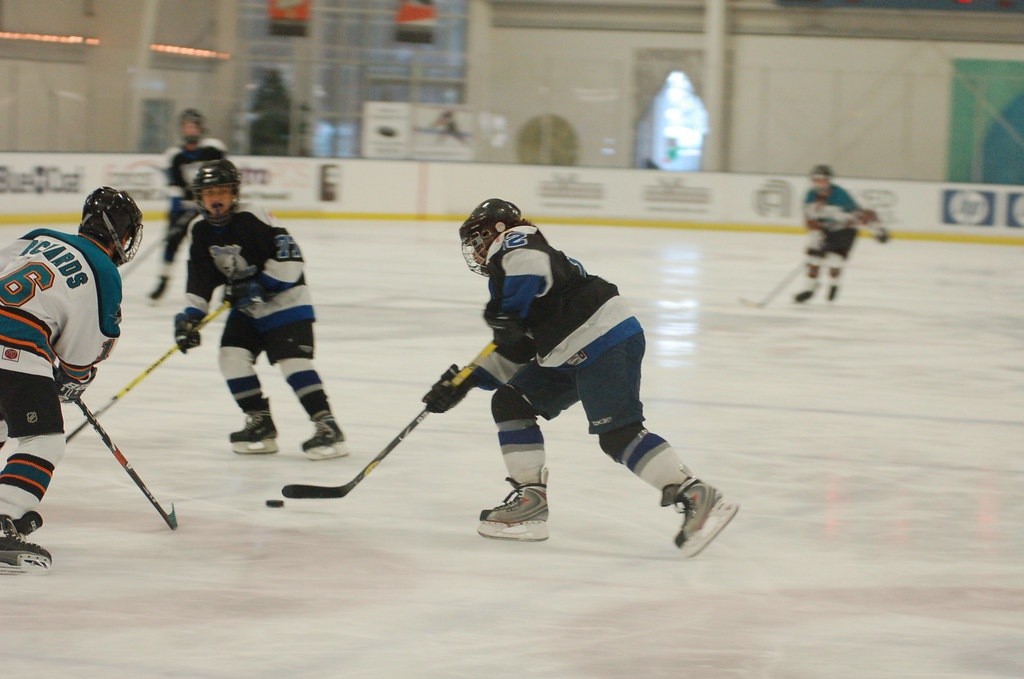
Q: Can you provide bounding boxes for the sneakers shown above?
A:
[231,398,279,455]
[0,510,52,574]
[150,278,169,301]
[660,467,741,557]
[302,413,351,460]
[476,466,548,542]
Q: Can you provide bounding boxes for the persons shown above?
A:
[175,159,350,460]
[147,107,227,301]
[792,166,887,303]
[246,69,291,157]
[423,198,741,558]
[0,186,144,576]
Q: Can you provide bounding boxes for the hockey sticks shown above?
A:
[52,362,179,532]
[119,211,196,275]
[280,341,499,500]
[65,300,230,443]
[742,259,808,308]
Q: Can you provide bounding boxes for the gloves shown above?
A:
[421,364,472,414]
[484,301,527,347]
[55,362,98,403]
[174,313,202,354]
[225,276,273,307]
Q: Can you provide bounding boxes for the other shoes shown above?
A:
[796,291,815,304]
[829,284,838,302]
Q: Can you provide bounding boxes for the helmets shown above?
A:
[811,164,834,180]
[459,199,522,278]
[178,108,202,127]
[191,159,241,228]
[79,186,142,267]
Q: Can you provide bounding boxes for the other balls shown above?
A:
[265,500,284,508]
